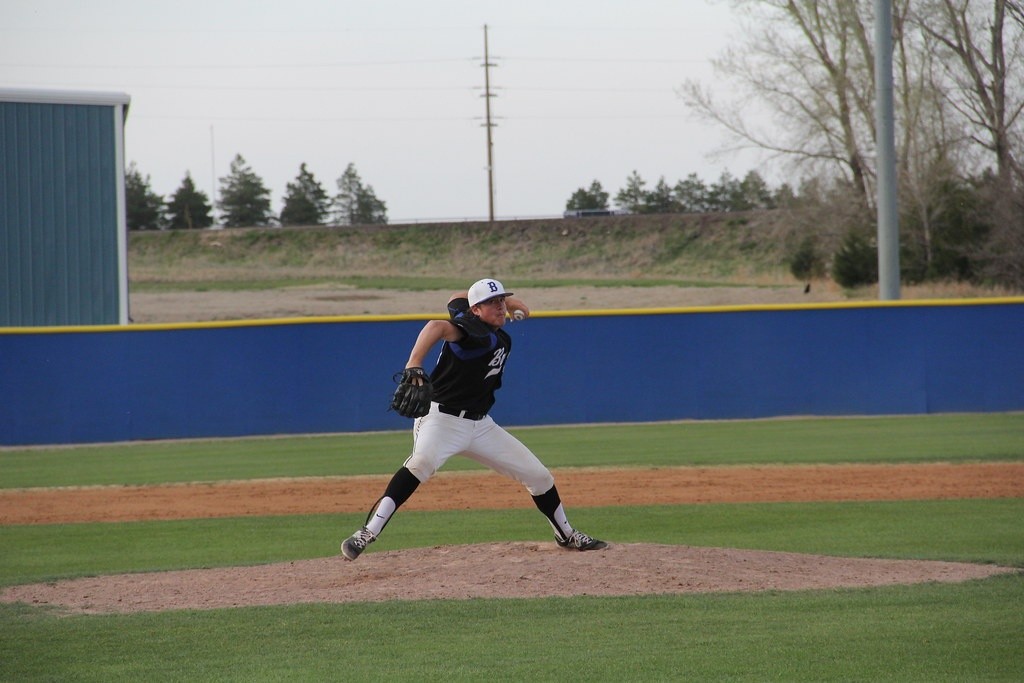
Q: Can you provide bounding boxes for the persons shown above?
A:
[339,279,610,562]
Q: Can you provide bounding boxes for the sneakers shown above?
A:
[341,525,379,561]
[554,528,610,552]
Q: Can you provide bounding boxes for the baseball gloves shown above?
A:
[385,366,434,419]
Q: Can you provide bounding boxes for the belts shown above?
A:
[439,405,484,421]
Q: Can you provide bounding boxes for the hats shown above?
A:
[467,278,515,308]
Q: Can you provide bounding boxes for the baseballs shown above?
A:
[514,309,524,321]
